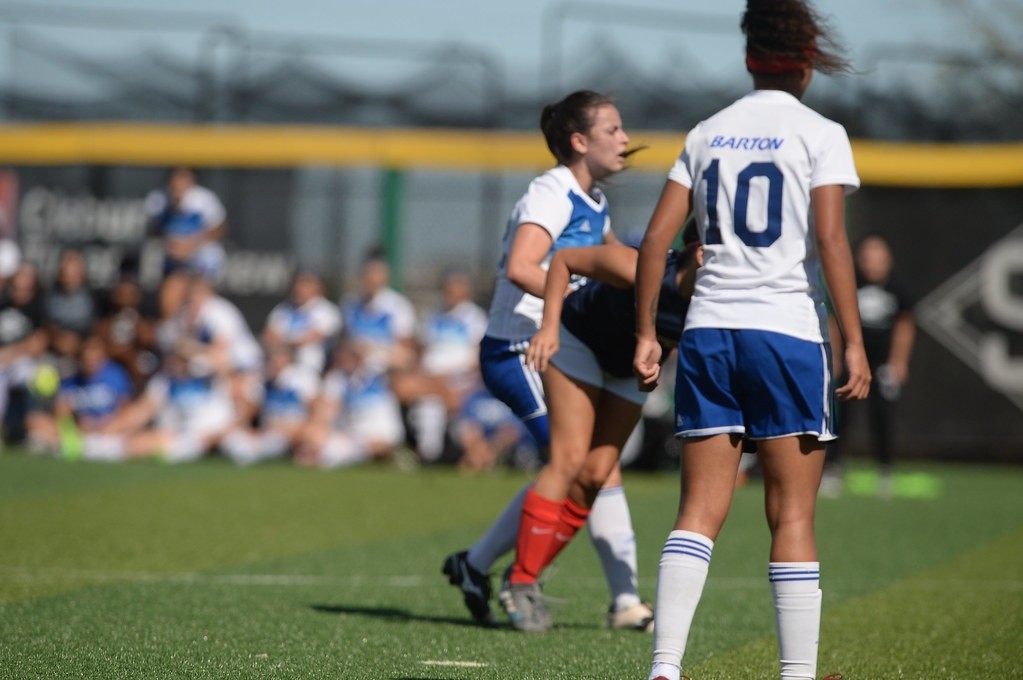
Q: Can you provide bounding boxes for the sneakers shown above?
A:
[442,550,502,629]
[499,562,570,633]
[608,599,655,634]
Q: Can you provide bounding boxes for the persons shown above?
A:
[817,228,914,502]
[0,165,842,499]
[441,90,658,632]
[635,0,872,680]
[500,219,704,632]
